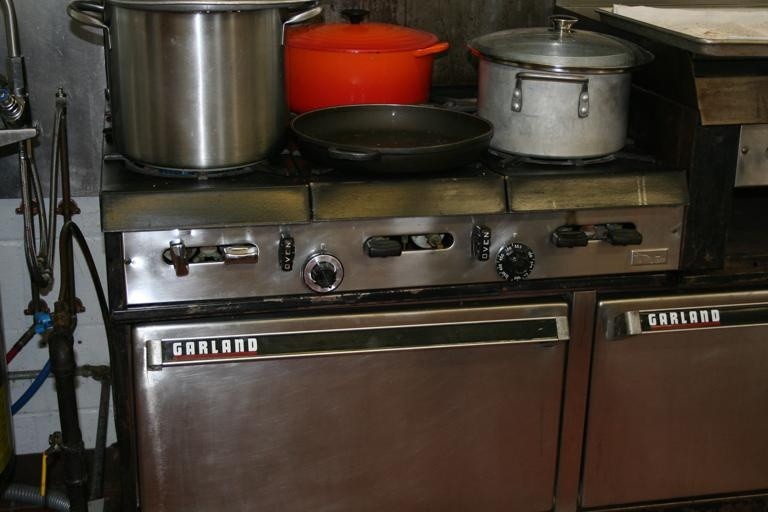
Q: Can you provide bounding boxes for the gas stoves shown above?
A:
[97,167,694,317]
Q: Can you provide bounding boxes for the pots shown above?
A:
[291,103,495,172]
[468,15,651,164]
[284,23,447,107]
[66,3,320,172]
